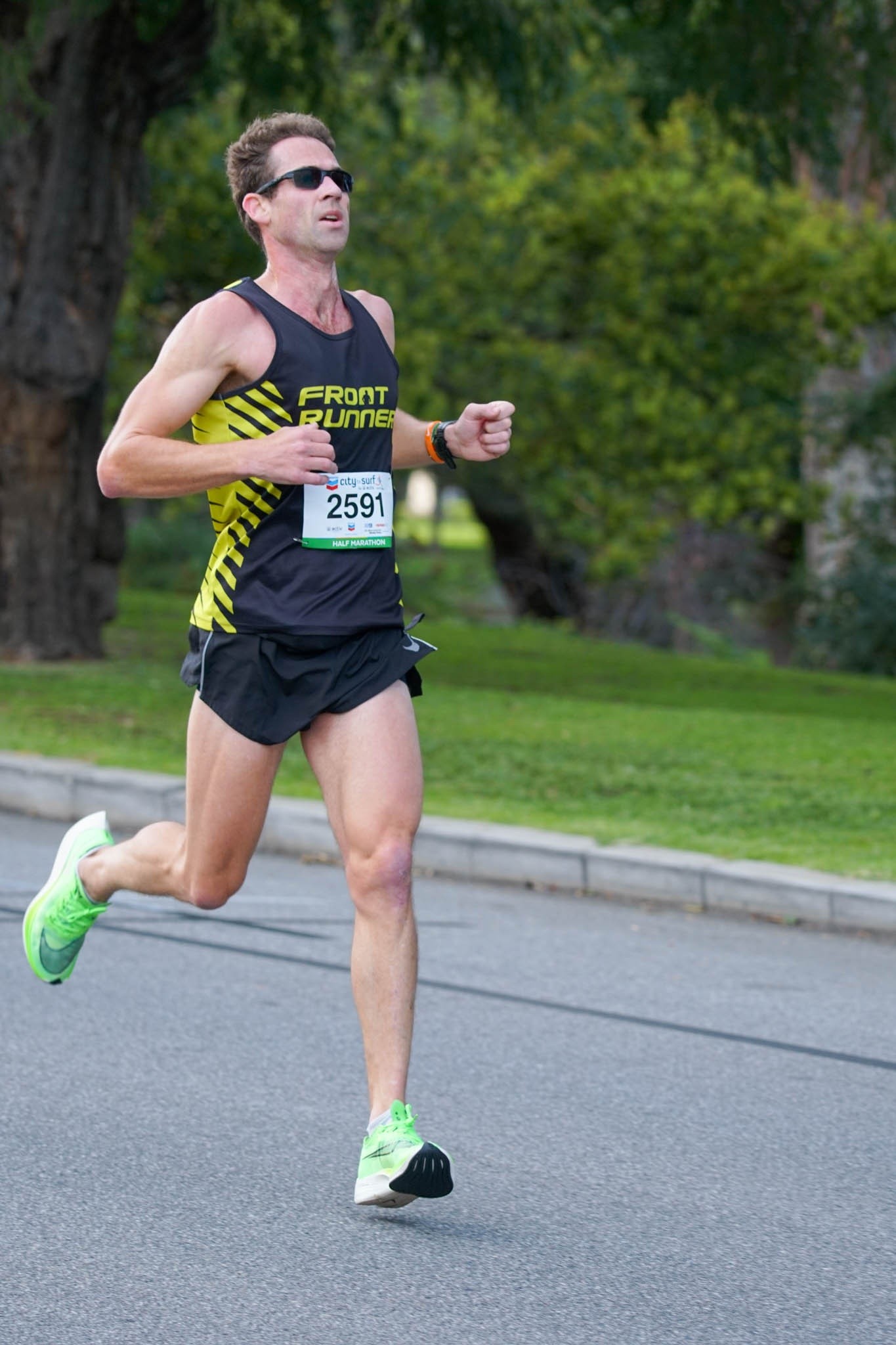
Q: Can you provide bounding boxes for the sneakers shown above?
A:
[21,811,116,986]
[353,1099,453,1209]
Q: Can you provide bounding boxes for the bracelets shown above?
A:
[424,420,456,468]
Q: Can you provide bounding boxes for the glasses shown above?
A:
[253,164,356,196]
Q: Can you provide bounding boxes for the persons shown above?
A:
[20,114,512,1209]
